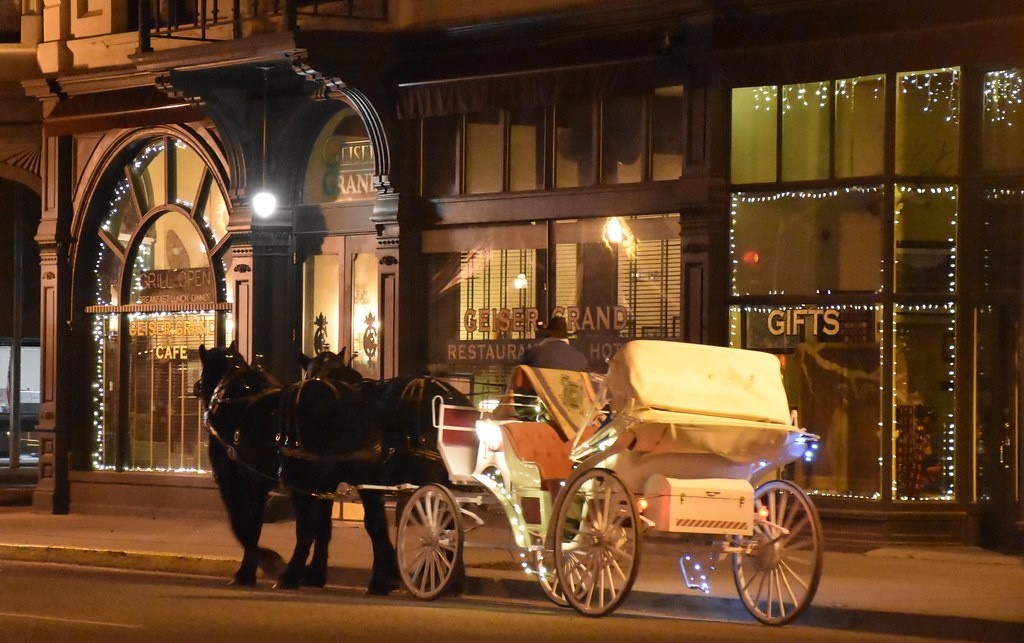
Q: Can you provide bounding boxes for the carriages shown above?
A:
[194,335,828,619]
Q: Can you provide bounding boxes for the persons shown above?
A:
[514,315,587,412]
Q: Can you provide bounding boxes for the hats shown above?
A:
[536,317,576,338]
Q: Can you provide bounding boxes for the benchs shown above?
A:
[488,364,610,421]
[569,340,793,495]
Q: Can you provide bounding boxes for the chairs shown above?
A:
[499,422,572,546]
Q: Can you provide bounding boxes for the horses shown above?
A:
[193,338,476,597]
